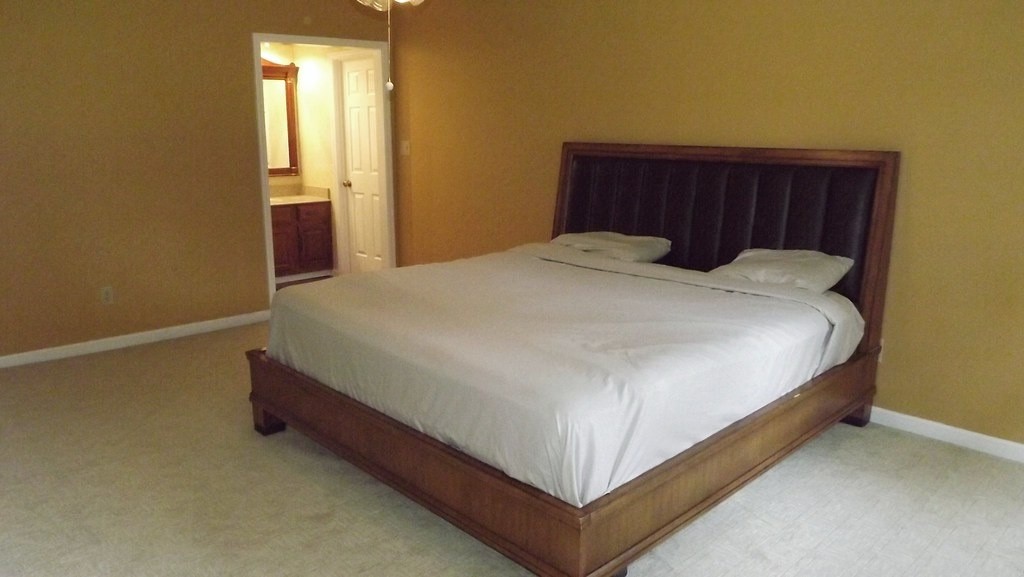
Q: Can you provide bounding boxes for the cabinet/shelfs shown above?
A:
[270,204,299,278]
[298,201,334,274]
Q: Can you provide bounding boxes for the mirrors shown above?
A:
[262,78,290,169]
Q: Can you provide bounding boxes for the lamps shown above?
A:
[356,0,425,91]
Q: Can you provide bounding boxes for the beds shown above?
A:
[245,240,867,577]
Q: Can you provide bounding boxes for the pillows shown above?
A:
[708,249,855,295]
[549,231,672,264]
